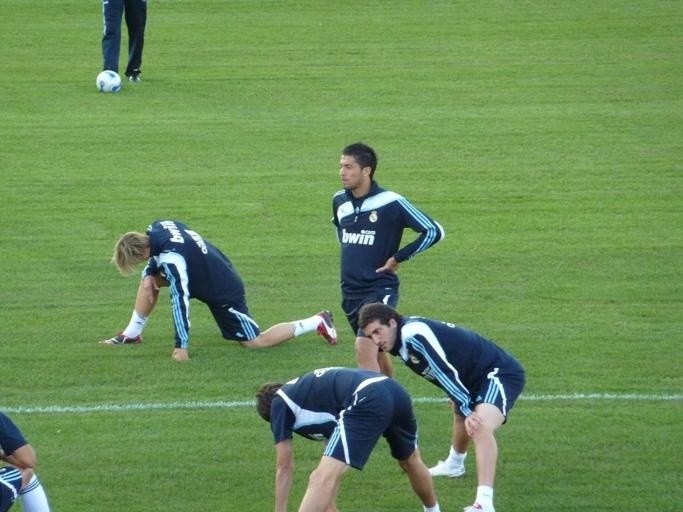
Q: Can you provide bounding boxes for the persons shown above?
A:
[99,220,338,361]
[101,1,147,83]
[0,413,48,512]
[254,367,441,512]
[330,144,445,377]
[358,302,525,512]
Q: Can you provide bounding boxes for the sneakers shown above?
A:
[316,310,337,345]
[129,69,141,81]
[463,504,494,512]
[98,334,141,344]
[429,460,465,478]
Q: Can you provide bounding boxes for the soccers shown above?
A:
[96,70,122,92]
